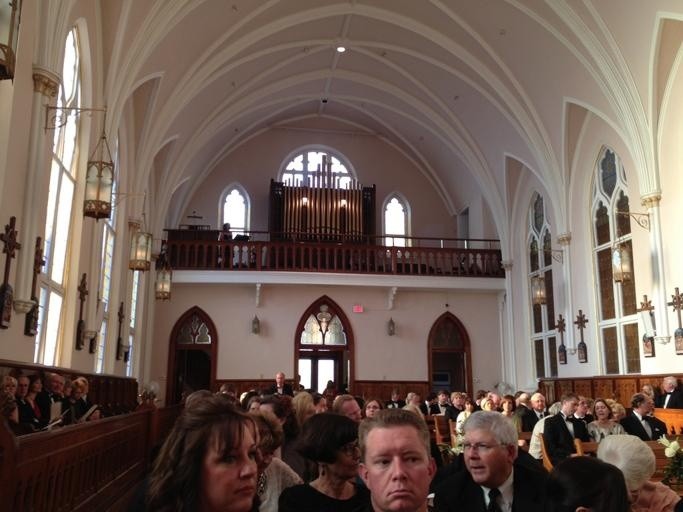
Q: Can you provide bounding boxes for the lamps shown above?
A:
[388,316,395,336]
[144,234,171,302]
[609,207,649,283]
[529,245,562,305]
[251,314,260,335]
[111,187,154,272]
[41,103,113,221]
[0,1,21,82]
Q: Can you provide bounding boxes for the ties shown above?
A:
[488,488,503,512]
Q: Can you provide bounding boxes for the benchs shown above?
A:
[423,415,682,512]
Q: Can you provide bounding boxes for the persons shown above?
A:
[131,373,683,512]
[0,373,101,437]
[218,224,234,267]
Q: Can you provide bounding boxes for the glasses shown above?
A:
[458,443,508,453]
[340,441,361,458]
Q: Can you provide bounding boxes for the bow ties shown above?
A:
[642,415,651,422]
[565,416,574,423]
[441,403,447,407]
[665,391,672,395]
